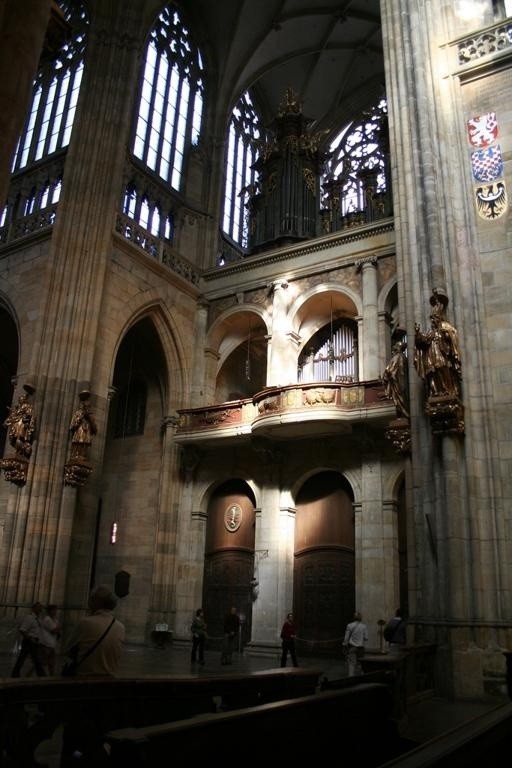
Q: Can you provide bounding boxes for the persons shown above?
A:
[343,610,369,657]
[3,394,36,457]
[384,606,409,645]
[70,399,100,462]
[280,612,299,667]
[62,583,128,674]
[382,340,410,419]
[413,303,466,397]
[188,607,208,665]
[36,603,64,675]
[219,606,242,665]
[8,600,49,676]
